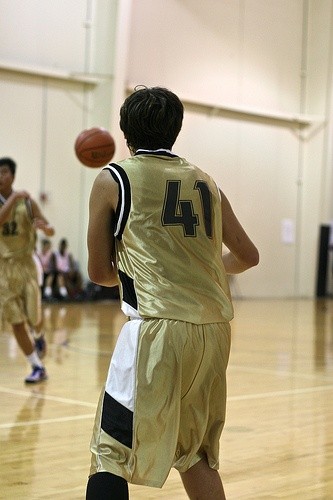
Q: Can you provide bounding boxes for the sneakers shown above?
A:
[35,331,46,359]
[26,366,45,382]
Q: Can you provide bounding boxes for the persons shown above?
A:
[0,156,56,382]
[38,239,84,302]
[86,87,258,500]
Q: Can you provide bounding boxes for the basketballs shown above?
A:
[73,128,116,168]
